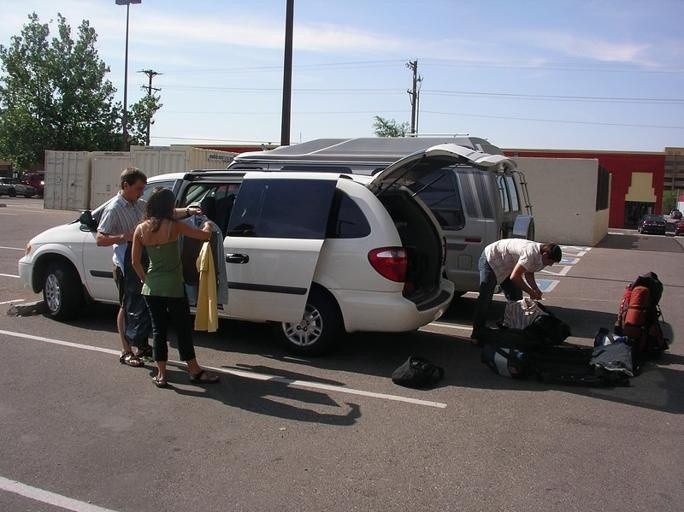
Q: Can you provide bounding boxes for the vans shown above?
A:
[19,144,516,357]
[227,136,535,299]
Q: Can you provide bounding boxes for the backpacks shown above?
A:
[475,300,633,389]
[614,272,669,376]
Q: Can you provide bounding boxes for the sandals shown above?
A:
[119,351,144,367]
[135,345,153,357]
[152,375,168,387]
[188,369,220,385]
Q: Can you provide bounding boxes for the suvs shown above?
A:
[21,171,44,197]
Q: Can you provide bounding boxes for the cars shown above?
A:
[670,208,684,234]
[0,177,36,198]
[638,214,667,235]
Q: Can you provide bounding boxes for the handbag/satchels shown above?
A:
[391,355,440,387]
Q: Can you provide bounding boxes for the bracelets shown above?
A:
[186,208,190,216]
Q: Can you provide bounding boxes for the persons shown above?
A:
[96,168,203,367]
[472,239,562,338]
[131,187,220,388]
